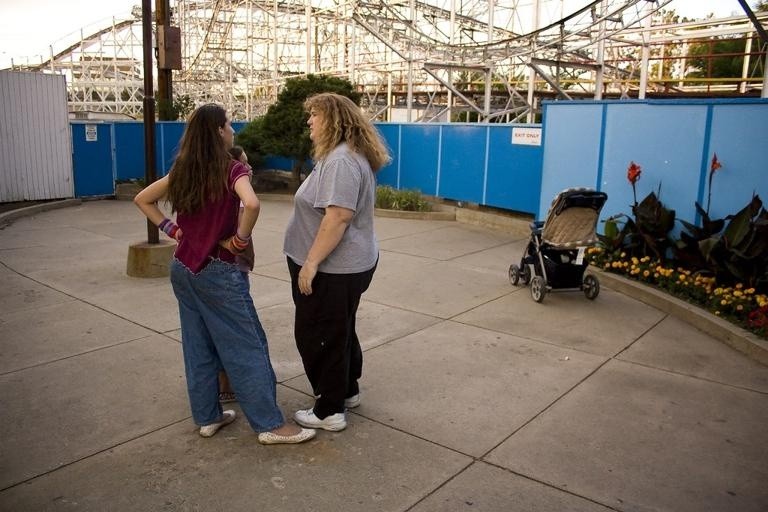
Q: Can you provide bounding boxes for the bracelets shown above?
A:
[228,232,252,255]
[154,219,179,239]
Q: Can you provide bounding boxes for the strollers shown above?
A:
[508,186,608,303]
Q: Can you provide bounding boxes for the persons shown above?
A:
[134,104,317,445]
[283,92,395,432]
[216,146,253,402]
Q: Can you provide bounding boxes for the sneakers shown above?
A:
[317,394,361,408]
[199,409,236,437]
[293,409,347,432]
[258,429,316,444]
[220,391,236,403]
